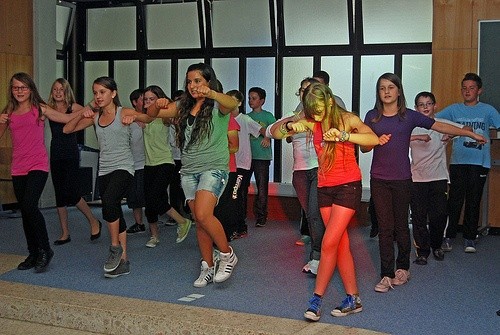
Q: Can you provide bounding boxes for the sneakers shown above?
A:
[302,260,319,274]
[104,242,123,272]
[176,219,192,243]
[233,230,247,238]
[35,248,54,272]
[375,276,394,292]
[370,224,378,238]
[228,231,238,241]
[413,256,427,264]
[296,235,311,245]
[194,258,214,287]
[126,223,146,235]
[441,237,453,252]
[18,255,36,270]
[304,296,322,321]
[330,295,363,317]
[214,246,238,283]
[165,218,177,226]
[392,269,410,285]
[104,259,130,278]
[464,239,476,252]
[431,244,444,261]
[256,220,266,227]
[145,237,160,247]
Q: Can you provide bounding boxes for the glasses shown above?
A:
[12,86,29,91]
[417,102,433,107]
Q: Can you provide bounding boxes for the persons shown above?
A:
[409,92,473,265]
[360,72,487,293]
[63,75,153,278]
[46,77,102,246]
[273,83,362,322]
[266,70,347,275]
[433,72,500,252]
[245,87,276,226]
[126,88,147,235]
[164,80,271,249]
[0,72,98,273]
[149,62,237,287]
[135,85,192,248]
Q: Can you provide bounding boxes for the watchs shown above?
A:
[334,134,341,142]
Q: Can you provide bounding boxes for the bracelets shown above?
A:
[286,120,294,131]
[211,89,217,99]
[341,130,351,143]
[89,102,95,110]
[279,123,289,136]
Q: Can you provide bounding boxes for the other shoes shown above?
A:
[54,234,71,245]
[90,220,102,241]
